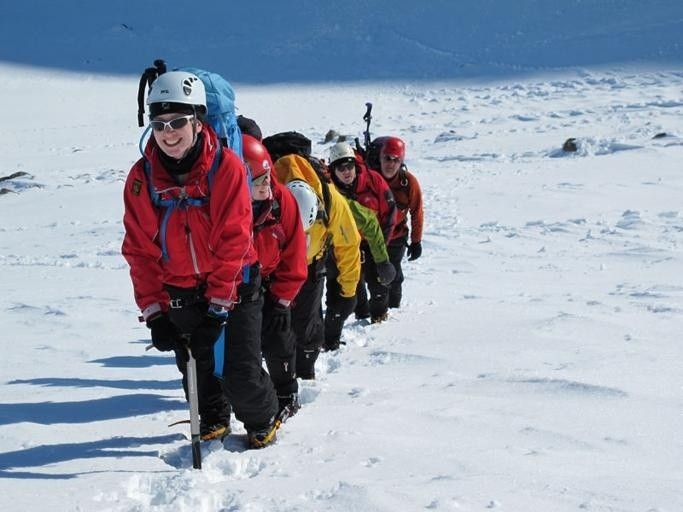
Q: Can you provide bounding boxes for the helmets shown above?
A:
[380,137,405,158]
[325,141,356,166]
[285,181,319,232]
[147,71,208,115]
[241,133,273,181]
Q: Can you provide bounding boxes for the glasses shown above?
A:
[150,114,194,130]
[252,171,269,187]
[337,162,355,173]
[383,155,400,164]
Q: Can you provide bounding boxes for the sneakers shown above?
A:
[278,393,300,422]
[199,424,231,440]
[248,416,280,449]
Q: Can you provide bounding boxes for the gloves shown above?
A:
[332,294,358,321]
[146,316,190,362]
[263,300,292,339]
[188,312,229,362]
[377,262,396,285]
[406,244,422,261]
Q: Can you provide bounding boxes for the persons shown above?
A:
[239,133,308,422]
[121,70,278,450]
[365,136,424,310]
[274,152,360,380]
[330,141,398,322]
[342,194,397,286]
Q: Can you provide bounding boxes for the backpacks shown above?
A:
[262,132,312,162]
[236,115,262,141]
[363,137,382,169]
[309,156,331,184]
[138,60,254,207]
